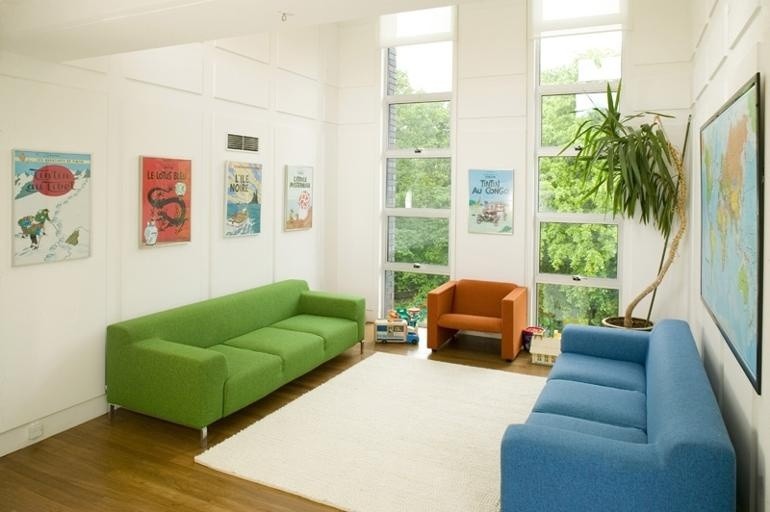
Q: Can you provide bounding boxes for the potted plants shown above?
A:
[553,71,691,336]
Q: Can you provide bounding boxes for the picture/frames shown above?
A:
[139,155,193,248]
[699,72,762,397]
[285,164,314,232]
[225,159,264,236]
[11,147,94,267]
[466,168,514,235]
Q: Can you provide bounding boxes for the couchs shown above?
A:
[103,278,368,446]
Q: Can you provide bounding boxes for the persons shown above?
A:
[18,209,51,249]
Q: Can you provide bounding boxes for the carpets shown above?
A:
[192,351,549,511]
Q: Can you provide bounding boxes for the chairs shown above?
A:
[499,319,737,511]
[427,278,530,364]
